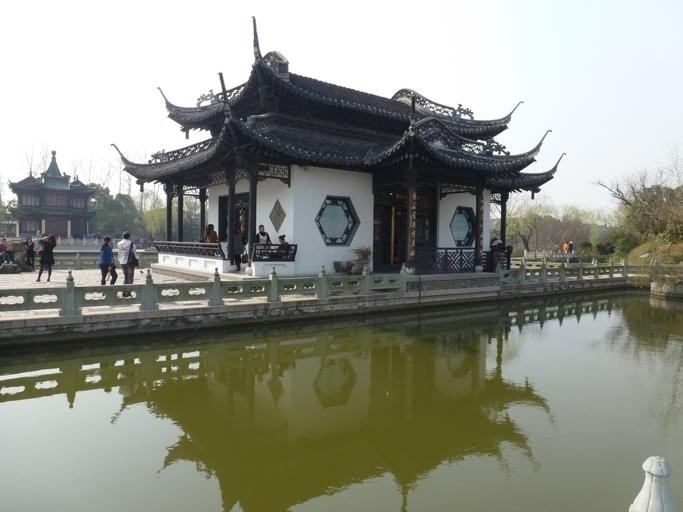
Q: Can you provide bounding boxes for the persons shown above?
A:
[278,235,290,260]
[99,237,117,296]
[24,238,35,269]
[233,221,243,272]
[117,231,137,298]
[36,233,56,282]
[204,224,219,256]
[559,239,576,255]
[0,238,13,265]
[256,225,271,259]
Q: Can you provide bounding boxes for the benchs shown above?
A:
[154,237,232,274]
[250,242,299,278]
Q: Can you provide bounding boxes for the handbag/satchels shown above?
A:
[110,263,116,269]
[241,253,248,263]
[128,251,138,267]
